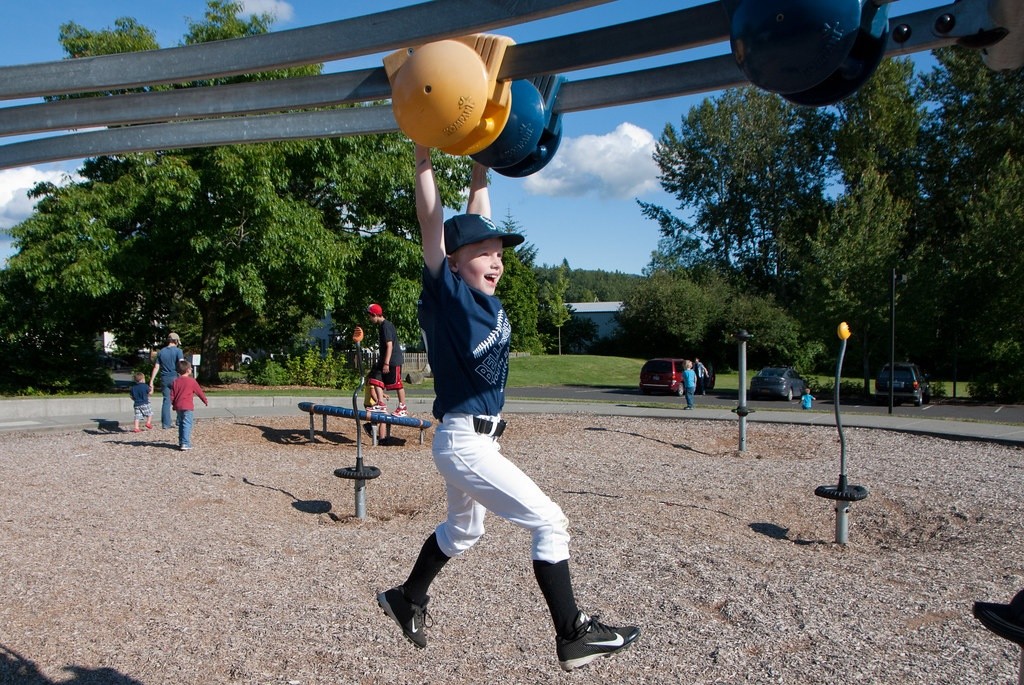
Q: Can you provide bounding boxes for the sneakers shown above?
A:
[378,437,393,446]
[376,586,433,649]
[365,401,387,413]
[555,609,641,672]
[363,422,373,438]
[391,403,409,417]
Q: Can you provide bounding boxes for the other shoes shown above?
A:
[684,406,693,410]
[181,444,192,449]
[133,428,141,432]
[145,422,153,429]
[162,423,175,428]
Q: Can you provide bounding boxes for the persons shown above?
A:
[130,371,153,432]
[363,302,409,444]
[679,359,697,409]
[796,388,816,409]
[170,359,209,450]
[693,357,709,395]
[376,142,643,673]
[149,332,185,429]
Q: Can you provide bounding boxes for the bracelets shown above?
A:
[384,362,390,365]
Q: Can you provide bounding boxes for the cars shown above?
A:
[750,367,806,401]
[639,359,715,397]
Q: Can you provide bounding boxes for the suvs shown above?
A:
[874,362,930,406]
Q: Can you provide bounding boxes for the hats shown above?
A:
[168,333,182,345]
[974,589,1024,647]
[444,214,525,256]
[366,304,383,315]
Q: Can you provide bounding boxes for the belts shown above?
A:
[438,413,507,437]
